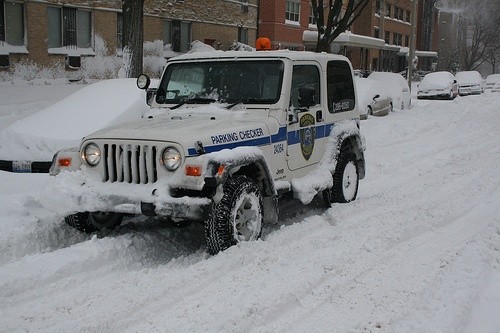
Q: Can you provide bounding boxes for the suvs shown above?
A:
[51,36,368,256]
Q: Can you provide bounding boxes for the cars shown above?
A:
[355,67,500,120]
